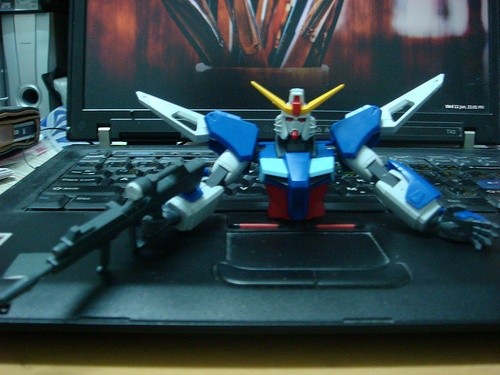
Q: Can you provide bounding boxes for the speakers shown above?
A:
[3,12,59,120]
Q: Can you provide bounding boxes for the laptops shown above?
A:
[0,0,500,334]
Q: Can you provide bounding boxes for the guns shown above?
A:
[1,155,210,315]
